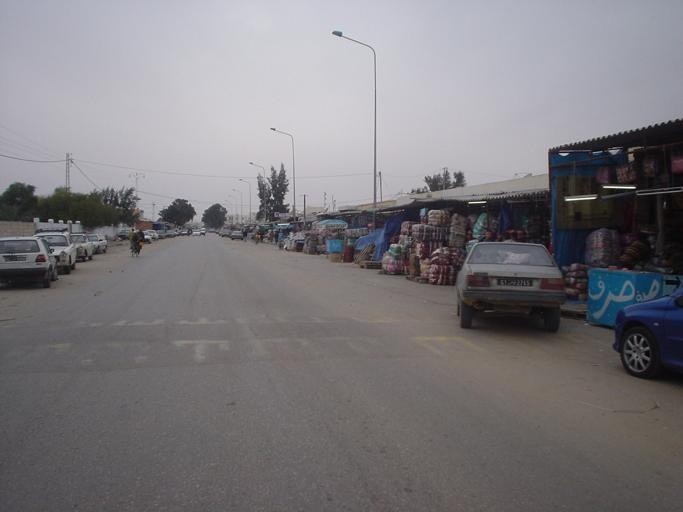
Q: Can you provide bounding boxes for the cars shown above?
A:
[611,282,682,380]
[118,227,247,243]
[454,240,566,334]
[0,229,108,290]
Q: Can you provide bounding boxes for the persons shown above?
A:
[240,226,281,246]
[127,227,144,258]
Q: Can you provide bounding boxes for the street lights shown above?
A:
[331,28,377,235]
[223,178,252,229]
[249,161,266,223]
[269,126,295,224]
